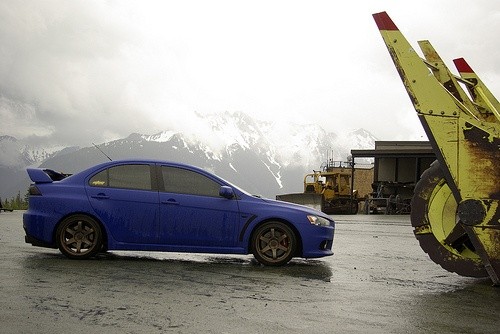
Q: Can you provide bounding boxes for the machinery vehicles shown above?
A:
[275,171,358,215]
[372,10,500,285]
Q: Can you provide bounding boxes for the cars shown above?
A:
[22,142,335,267]
